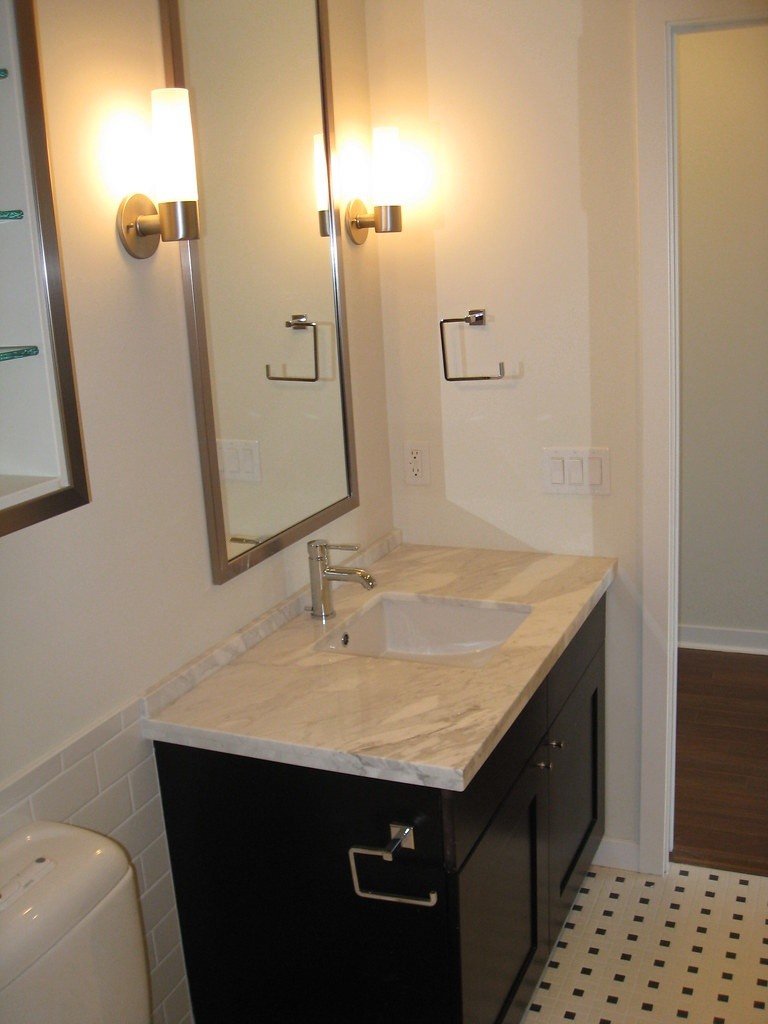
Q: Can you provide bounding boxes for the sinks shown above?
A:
[306,591,536,665]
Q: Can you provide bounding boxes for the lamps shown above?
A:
[344,130,401,243]
[116,88,199,258]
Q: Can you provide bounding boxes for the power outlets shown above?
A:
[403,440,429,487]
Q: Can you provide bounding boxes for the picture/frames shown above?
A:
[0,0,91,536]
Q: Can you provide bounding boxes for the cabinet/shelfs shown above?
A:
[152,594,607,1024]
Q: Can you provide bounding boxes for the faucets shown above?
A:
[229,536,261,545]
[304,539,377,620]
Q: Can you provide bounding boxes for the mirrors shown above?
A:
[159,0,361,586]
